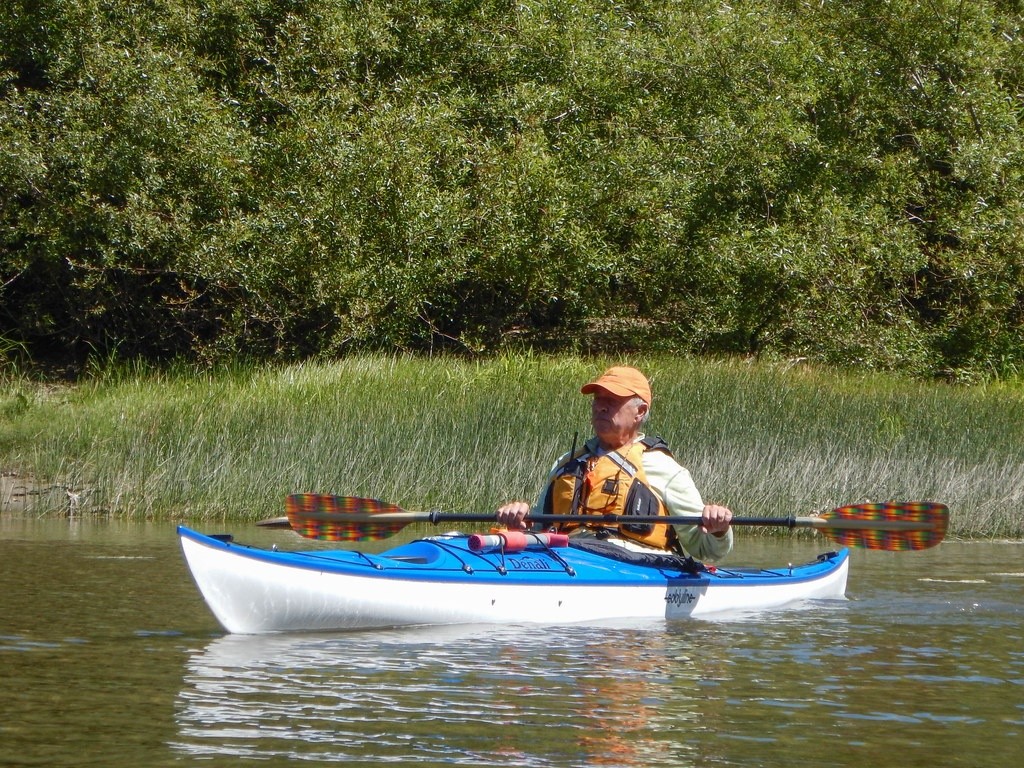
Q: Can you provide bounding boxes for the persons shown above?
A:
[497,366,733,563]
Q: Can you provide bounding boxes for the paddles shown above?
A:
[283,492,951,552]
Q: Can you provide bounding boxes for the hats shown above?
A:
[581,367,651,407]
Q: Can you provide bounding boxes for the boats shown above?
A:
[175,523,851,636]
